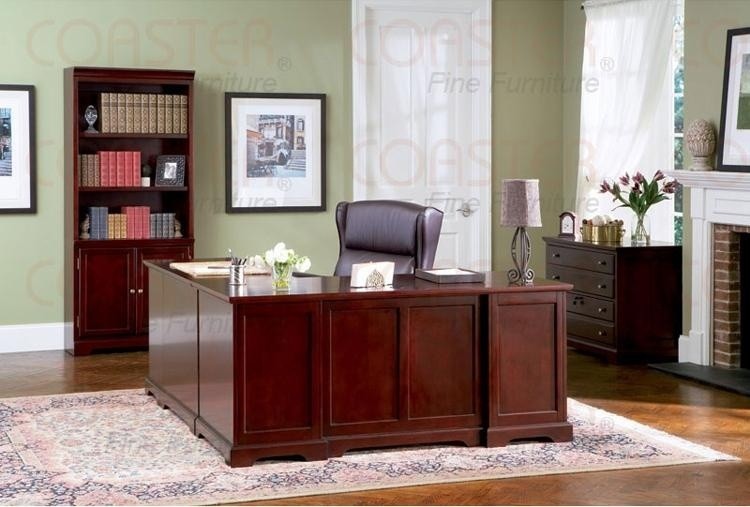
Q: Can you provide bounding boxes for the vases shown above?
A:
[272,264,293,290]
[629,218,650,243]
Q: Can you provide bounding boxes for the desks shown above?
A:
[143,258,575,467]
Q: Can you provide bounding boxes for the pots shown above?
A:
[580,224,626,242]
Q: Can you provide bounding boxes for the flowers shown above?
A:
[264,242,309,284]
[597,170,679,221]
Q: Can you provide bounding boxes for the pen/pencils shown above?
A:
[207,267,230,269]
[230,257,246,266]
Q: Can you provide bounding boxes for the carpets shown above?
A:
[1,393,743,506]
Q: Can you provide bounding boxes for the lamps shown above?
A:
[499,178,545,283]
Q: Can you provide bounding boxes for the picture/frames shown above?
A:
[224,90,326,213]
[715,28,749,171]
[0,83,36,214]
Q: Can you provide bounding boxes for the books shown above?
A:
[76,151,140,187]
[97,91,187,135]
[87,204,176,239]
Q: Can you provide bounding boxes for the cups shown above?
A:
[229,265,246,286]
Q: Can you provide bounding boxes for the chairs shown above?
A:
[333,200,444,277]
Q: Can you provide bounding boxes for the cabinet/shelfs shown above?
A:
[540,230,682,367]
[63,65,196,358]
